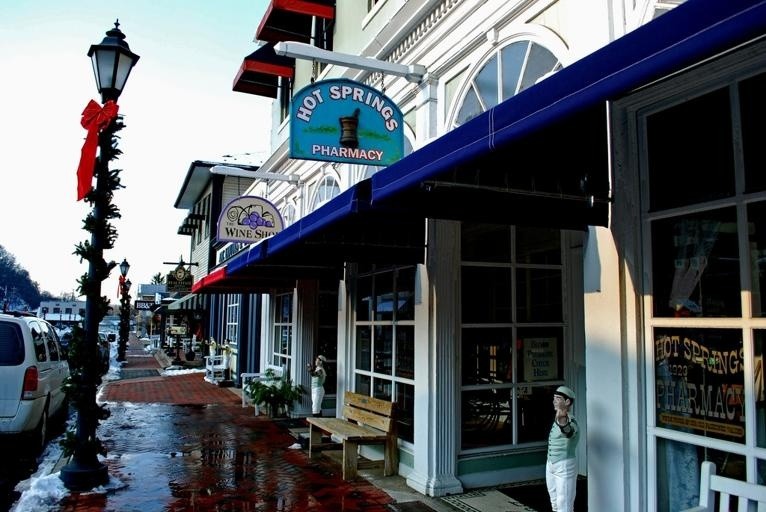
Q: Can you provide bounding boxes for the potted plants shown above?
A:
[242,367,309,421]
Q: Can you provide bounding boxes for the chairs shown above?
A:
[203,353,234,384]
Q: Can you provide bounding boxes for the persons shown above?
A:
[546,386,580,512]
[305,355,327,417]
[205,336,217,365]
[217,338,231,380]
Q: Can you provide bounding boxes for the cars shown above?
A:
[0,311,72,454]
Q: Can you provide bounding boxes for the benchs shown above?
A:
[305,389,401,482]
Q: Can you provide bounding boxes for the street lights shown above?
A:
[118,257,132,360]
[59,14,141,492]
